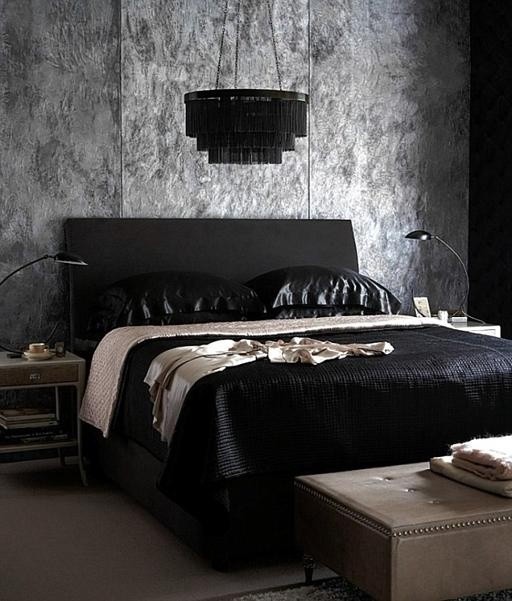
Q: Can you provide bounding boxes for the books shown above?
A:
[1,408,74,454]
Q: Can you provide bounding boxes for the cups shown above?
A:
[29,342,49,353]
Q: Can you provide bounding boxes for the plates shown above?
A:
[23,352,56,360]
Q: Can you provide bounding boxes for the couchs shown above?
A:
[293,461,511,601]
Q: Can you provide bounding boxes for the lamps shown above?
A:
[0,252,89,287]
[185,1,309,165]
[404,230,488,325]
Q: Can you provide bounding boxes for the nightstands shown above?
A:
[1,349,91,489]
[450,322,502,339]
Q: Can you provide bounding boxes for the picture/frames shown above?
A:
[412,295,432,318]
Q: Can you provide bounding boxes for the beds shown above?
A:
[63,216,510,573]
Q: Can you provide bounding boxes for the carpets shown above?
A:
[200,576,512,600]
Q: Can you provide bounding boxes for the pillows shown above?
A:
[245,265,402,319]
[87,269,267,331]
